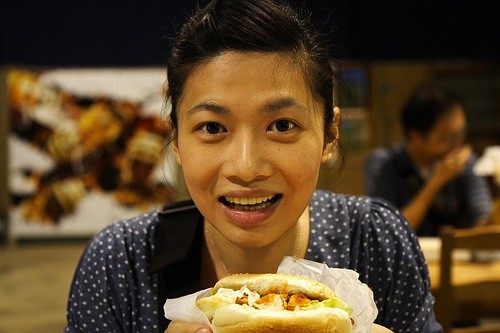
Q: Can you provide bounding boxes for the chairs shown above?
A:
[432,226,500,333]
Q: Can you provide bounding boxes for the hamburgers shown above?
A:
[198,272,353,333]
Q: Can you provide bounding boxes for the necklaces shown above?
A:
[203,217,303,276]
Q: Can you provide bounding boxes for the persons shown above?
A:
[66,1,444,331]
[367,81,498,237]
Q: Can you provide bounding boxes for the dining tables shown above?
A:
[418,236,500,322]
